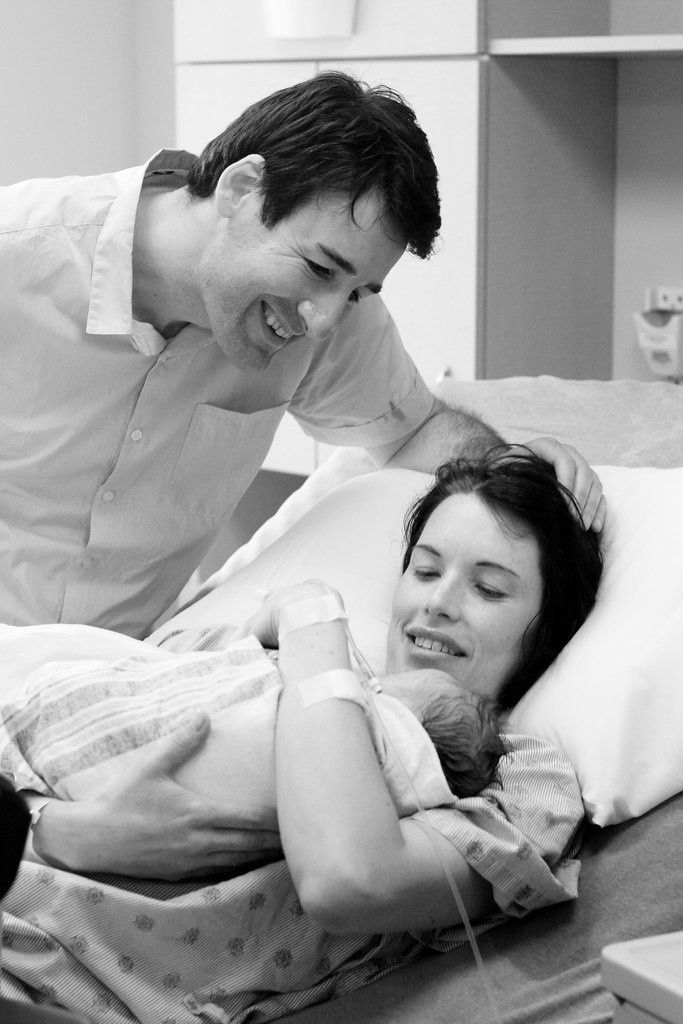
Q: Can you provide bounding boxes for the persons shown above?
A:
[0,647,507,870]
[0,73,609,906]
[0,440,607,1024]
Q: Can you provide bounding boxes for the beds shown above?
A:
[2,376,682,1023]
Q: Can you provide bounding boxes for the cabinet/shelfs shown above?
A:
[169,1,481,380]
[478,1,680,382]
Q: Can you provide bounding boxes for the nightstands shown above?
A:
[598,930,680,1023]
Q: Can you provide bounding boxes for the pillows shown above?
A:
[146,462,681,829]
